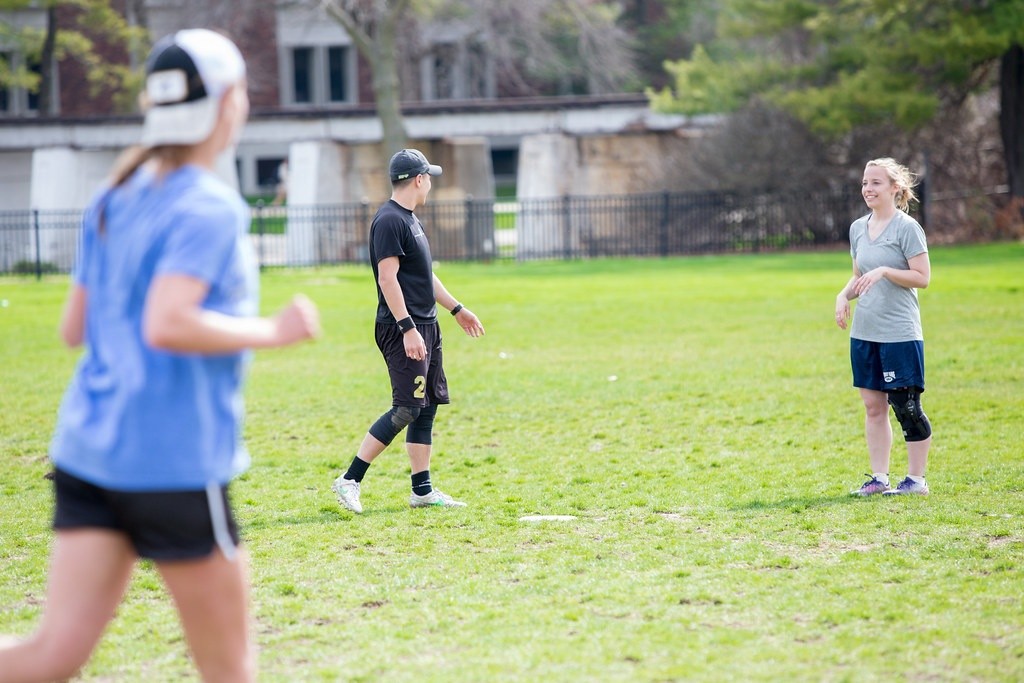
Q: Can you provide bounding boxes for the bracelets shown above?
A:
[396,315,417,334]
[449,303,465,317]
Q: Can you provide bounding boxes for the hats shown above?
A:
[389,148,442,182]
[140,26,247,150]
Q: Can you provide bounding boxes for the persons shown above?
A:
[330,147,486,514]
[835,157,931,496]
[0,27,321,683]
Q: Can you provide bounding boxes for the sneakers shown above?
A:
[332,470,364,514]
[850,472,892,496]
[883,475,931,496]
[409,486,467,508]
